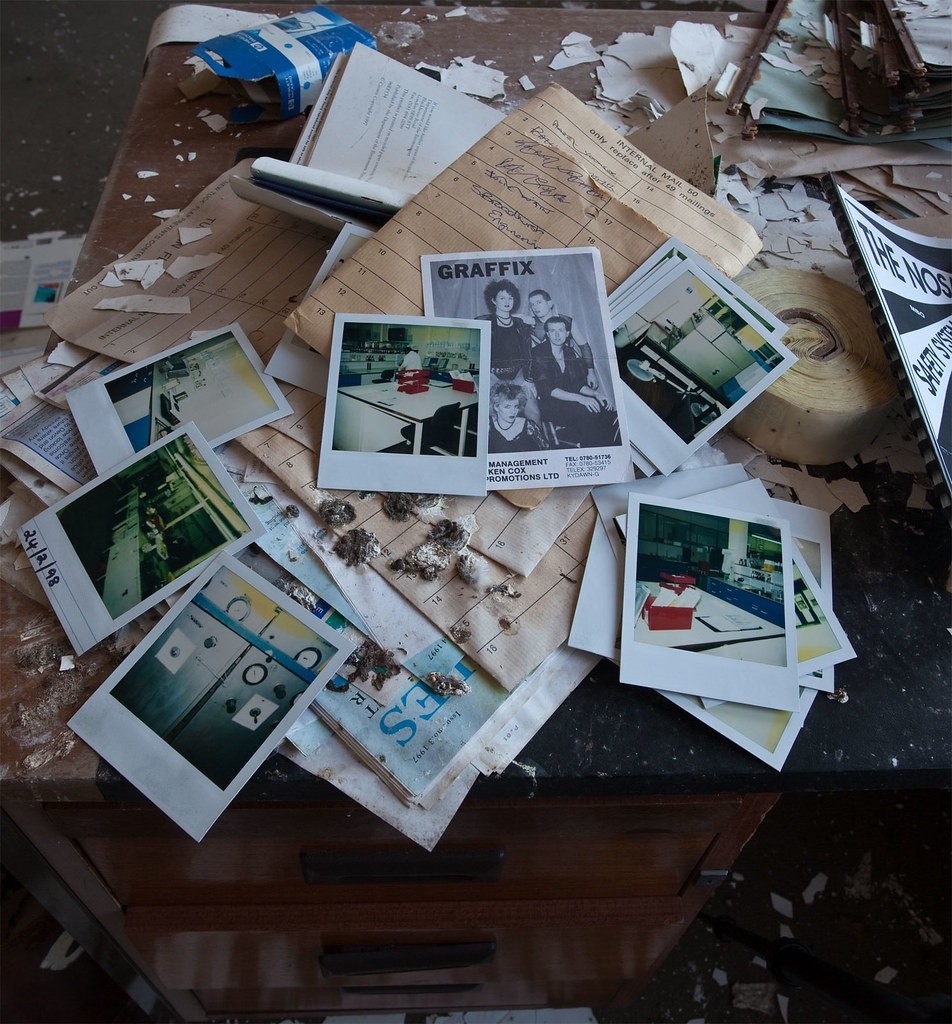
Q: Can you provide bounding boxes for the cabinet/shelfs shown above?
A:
[331,342,479,452]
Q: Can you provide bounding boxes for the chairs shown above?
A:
[401,402,461,454]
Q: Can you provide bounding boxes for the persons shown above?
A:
[393,277,624,468]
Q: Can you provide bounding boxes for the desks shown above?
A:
[0,2,952,1024]
